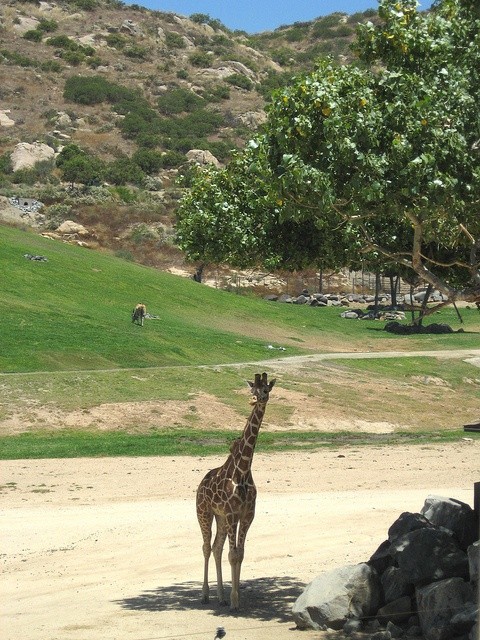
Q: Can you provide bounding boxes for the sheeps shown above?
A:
[132,303,146,326]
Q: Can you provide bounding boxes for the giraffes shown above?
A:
[195,373,276,613]
